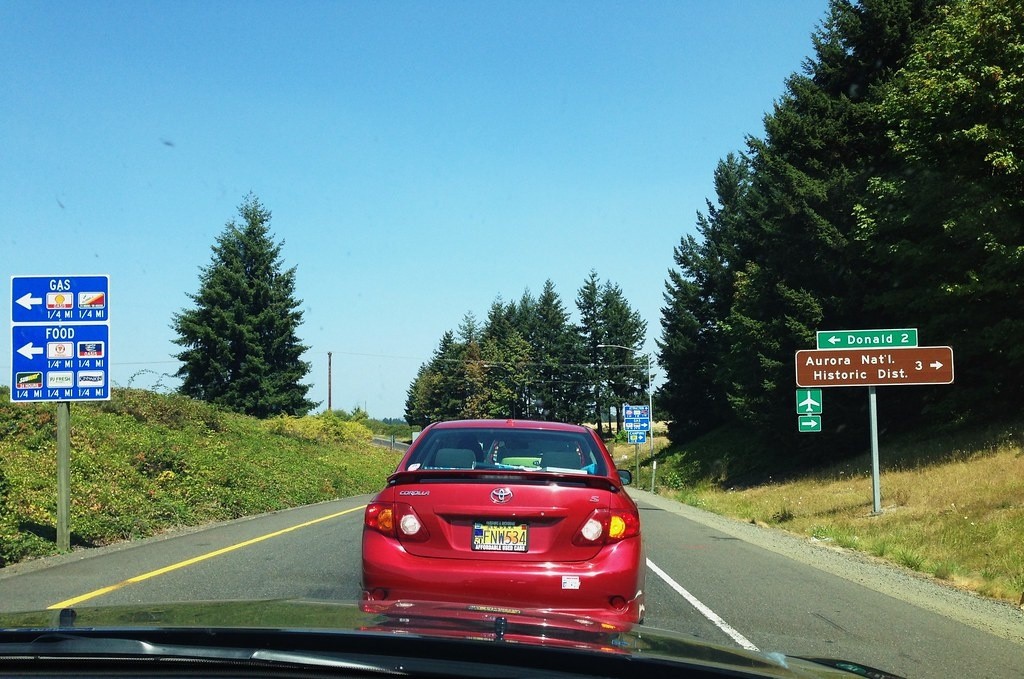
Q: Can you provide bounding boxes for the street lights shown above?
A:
[596,344,656,492]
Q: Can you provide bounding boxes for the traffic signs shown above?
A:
[13,276,109,321]
[798,349,954,385]
[13,326,110,401]
[817,328,920,348]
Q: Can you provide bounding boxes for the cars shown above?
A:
[359,416,647,626]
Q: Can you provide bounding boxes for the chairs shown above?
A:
[540,440,581,469]
[435,448,477,470]
[429,435,484,469]
